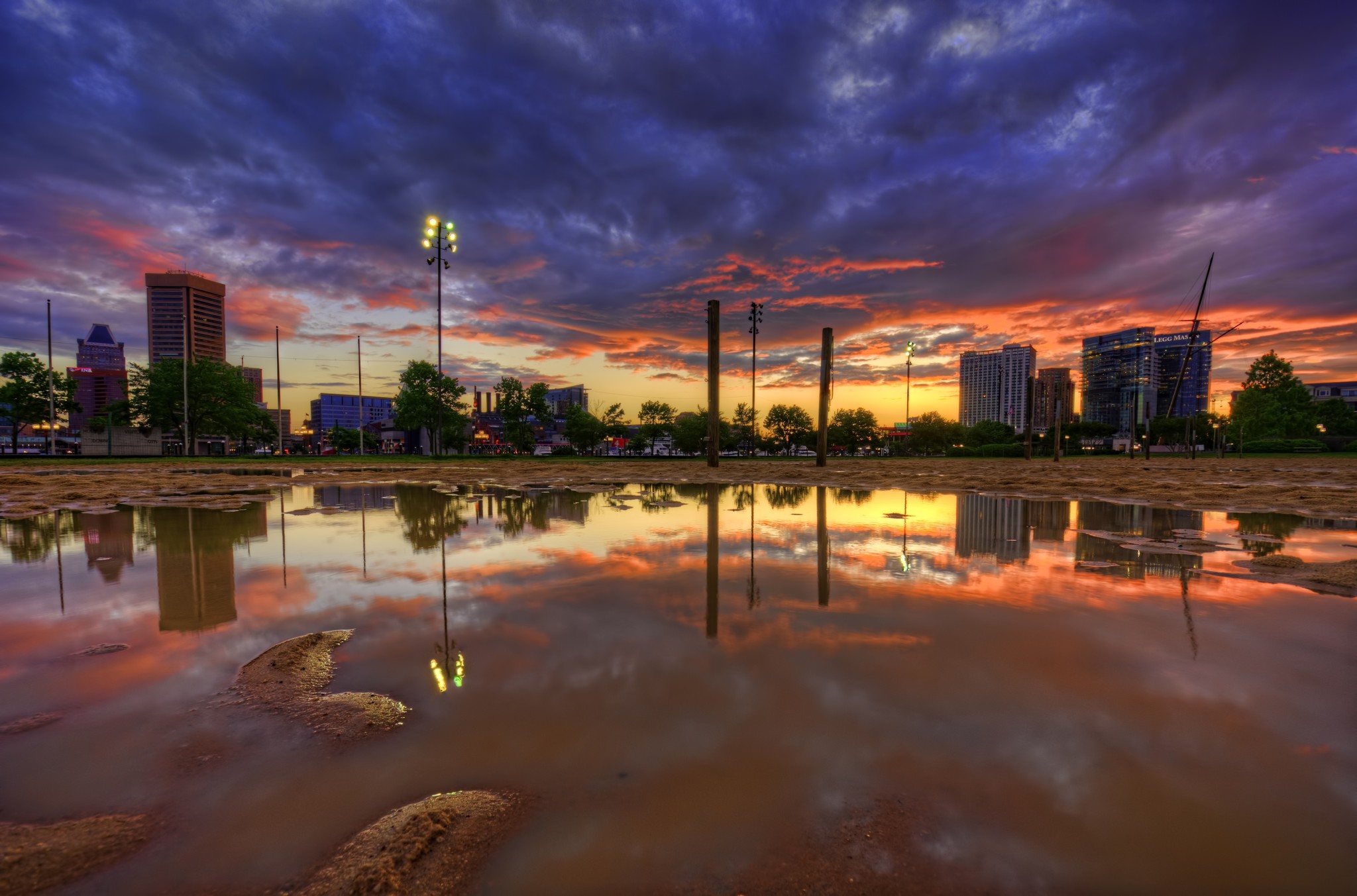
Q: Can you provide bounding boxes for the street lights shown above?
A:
[422,218,456,455]
[1065,435,1069,456]
[748,302,763,458]
[430,491,465,692]
[997,366,1005,422]
[1040,434,1044,442]
[905,341,916,456]
